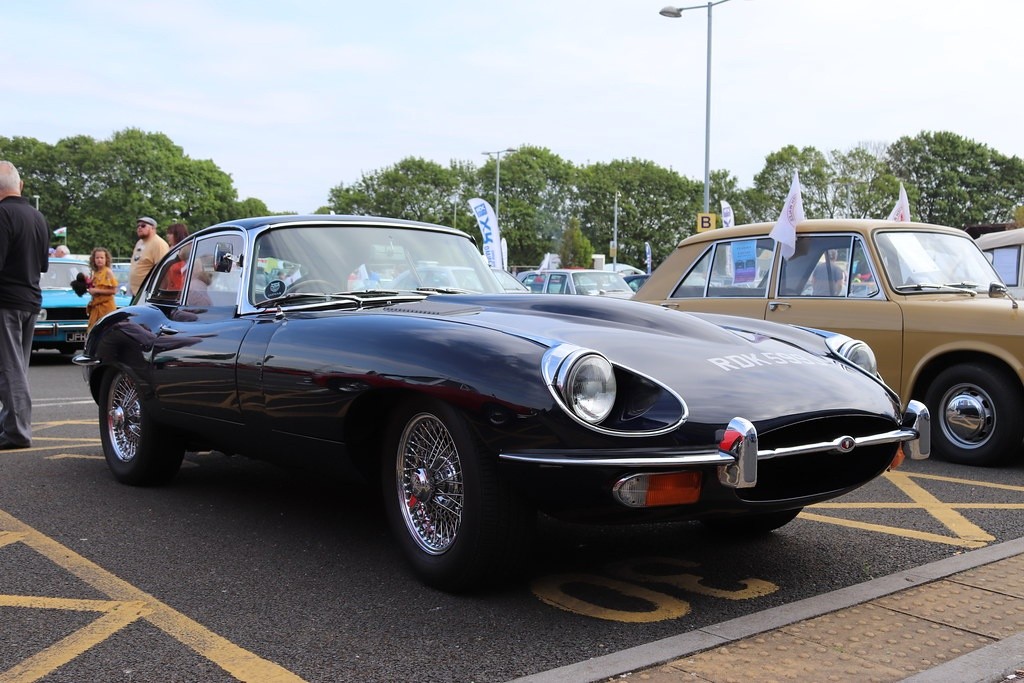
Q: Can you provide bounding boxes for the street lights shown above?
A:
[658,0,728,212]
[33,196,40,211]
[614,190,622,271]
[481,148,518,222]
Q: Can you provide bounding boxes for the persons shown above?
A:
[85,246,119,340]
[855,255,874,281]
[129,217,170,304]
[352,249,385,290]
[49,244,70,257]
[0,160,48,450]
[158,223,189,300]
[178,243,213,306]
[812,248,843,297]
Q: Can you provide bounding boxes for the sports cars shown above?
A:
[72,214,932,599]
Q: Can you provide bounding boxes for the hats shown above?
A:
[137,217,157,227]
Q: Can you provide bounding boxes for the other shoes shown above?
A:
[0,436,24,449]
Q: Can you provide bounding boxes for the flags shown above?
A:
[768,172,804,262]
[54,226,67,237]
[886,182,910,222]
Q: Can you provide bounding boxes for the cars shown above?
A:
[111,263,133,297]
[939,228,1024,299]
[32,259,134,355]
[514,267,637,301]
[628,219,1024,466]
[386,265,532,294]
[611,275,650,292]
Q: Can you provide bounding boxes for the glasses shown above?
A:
[137,224,151,228]
